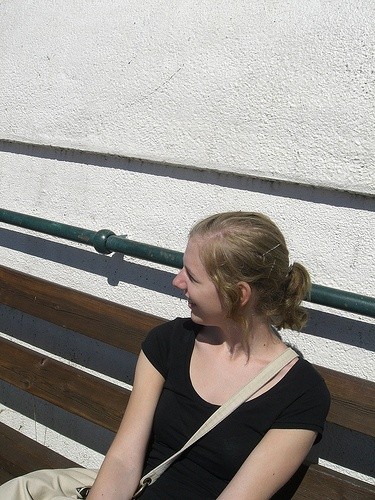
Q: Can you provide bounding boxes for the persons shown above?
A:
[85,212,331,500]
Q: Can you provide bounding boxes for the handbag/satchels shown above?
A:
[0,467,100,500]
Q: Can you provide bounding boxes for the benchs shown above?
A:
[1,264,374,500]
